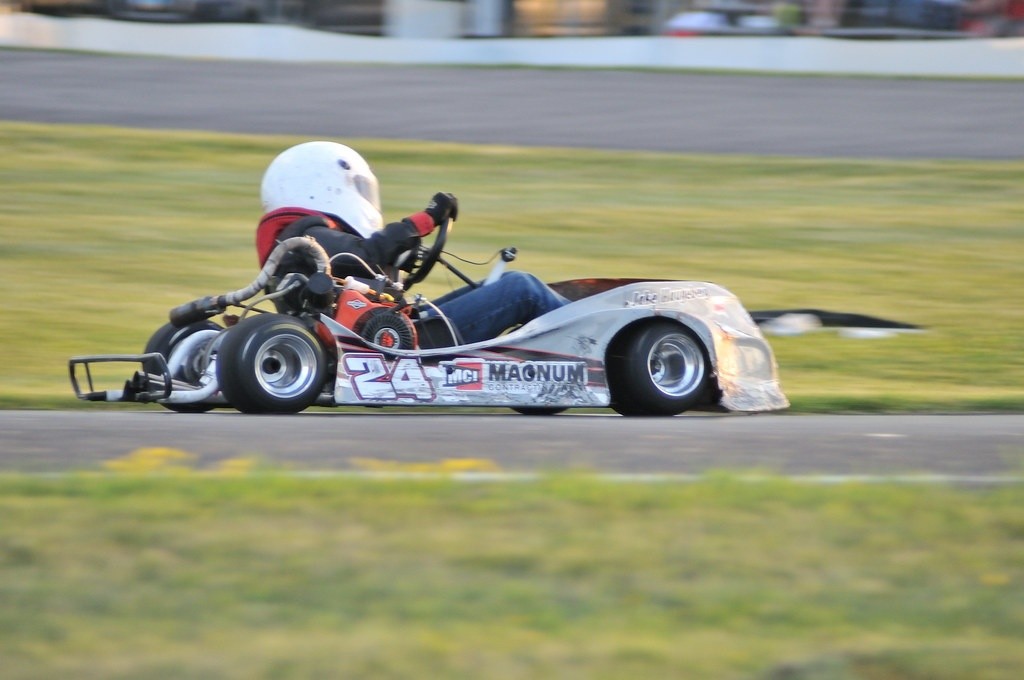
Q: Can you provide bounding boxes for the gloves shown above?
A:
[425,192,458,226]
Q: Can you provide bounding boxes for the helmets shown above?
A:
[260,141,384,240]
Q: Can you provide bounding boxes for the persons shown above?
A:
[257,141,571,350]
[613,0,1024,40]
